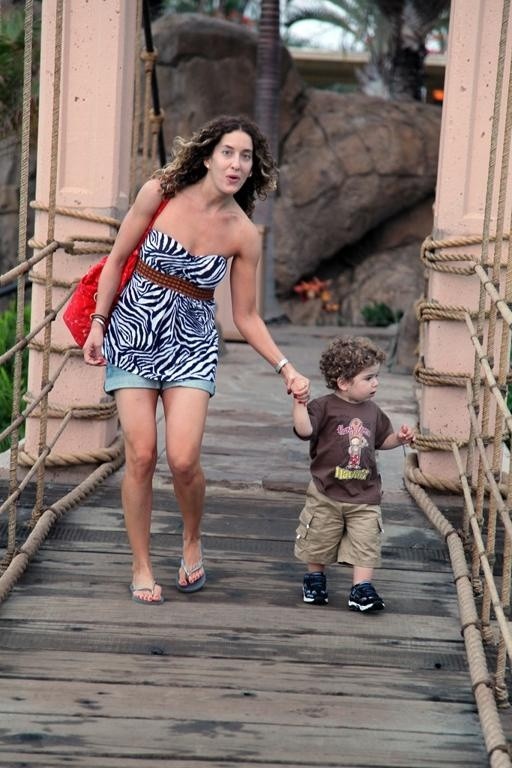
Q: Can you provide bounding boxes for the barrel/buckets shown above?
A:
[130,580,165,604]
[175,544,207,594]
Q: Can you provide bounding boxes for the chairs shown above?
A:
[301,572,329,606]
[348,581,386,613]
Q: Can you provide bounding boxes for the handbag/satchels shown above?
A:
[275,359,289,374]
[90,313,107,329]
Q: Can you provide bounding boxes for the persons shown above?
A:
[82,113,313,606]
[292,334,417,613]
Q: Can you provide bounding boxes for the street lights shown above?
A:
[62,189,173,351]
